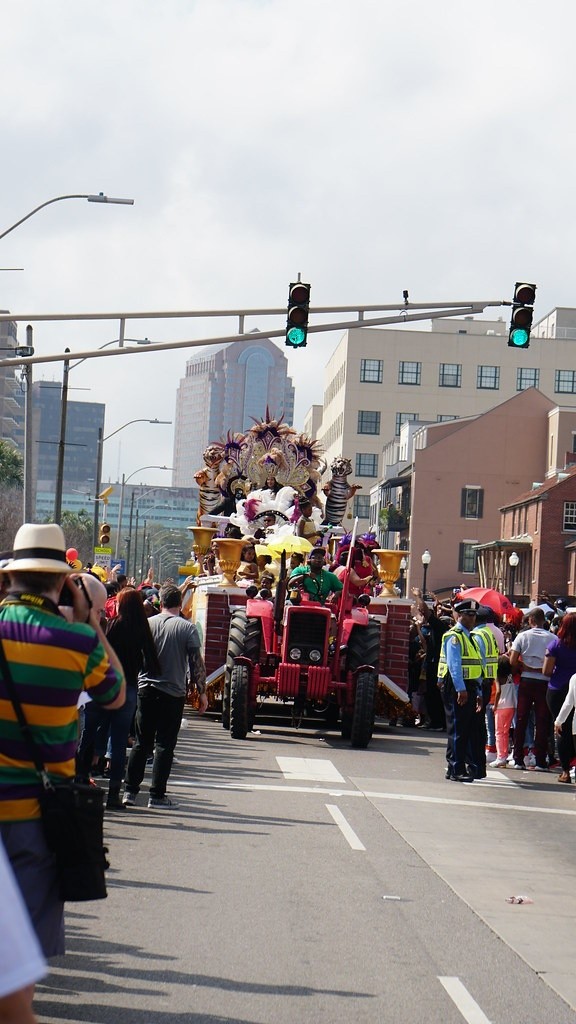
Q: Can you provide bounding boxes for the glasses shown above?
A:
[263,519,271,522]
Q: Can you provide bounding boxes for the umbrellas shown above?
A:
[455,587,513,622]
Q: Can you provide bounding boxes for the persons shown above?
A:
[204,474,374,605]
[388,583,576,783]
[0,524,208,1024]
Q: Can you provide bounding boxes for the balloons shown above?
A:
[65,549,82,571]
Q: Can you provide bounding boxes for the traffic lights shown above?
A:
[99,524,110,544]
[507,281,537,351]
[285,282,309,347]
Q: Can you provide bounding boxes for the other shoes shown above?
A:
[535,766,550,771]
[485,750,560,769]
[416,718,443,731]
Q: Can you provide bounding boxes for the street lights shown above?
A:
[140,517,174,586]
[133,505,178,584]
[421,549,433,601]
[54,337,162,524]
[93,418,173,569]
[509,551,519,608]
[400,556,409,599]
[125,487,182,579]
[145,528,183,581]
[113,466,174,561]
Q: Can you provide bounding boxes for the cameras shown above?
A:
[59,579,81,606]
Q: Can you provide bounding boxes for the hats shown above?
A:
[1,523,73,572]
[309,548,326,559]
[455,601,480,615]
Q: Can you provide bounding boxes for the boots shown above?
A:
[106,789,126,810]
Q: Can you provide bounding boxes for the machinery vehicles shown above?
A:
[220,518,385,749]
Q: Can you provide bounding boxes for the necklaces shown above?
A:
[310,570,323,596]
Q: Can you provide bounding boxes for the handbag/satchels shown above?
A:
[40,772,107,902]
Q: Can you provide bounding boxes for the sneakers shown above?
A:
[147,795,179,810]
[450,773,473,782]
[558,774,571,782]
[122,792,136,805]
[445,774,450,779]
